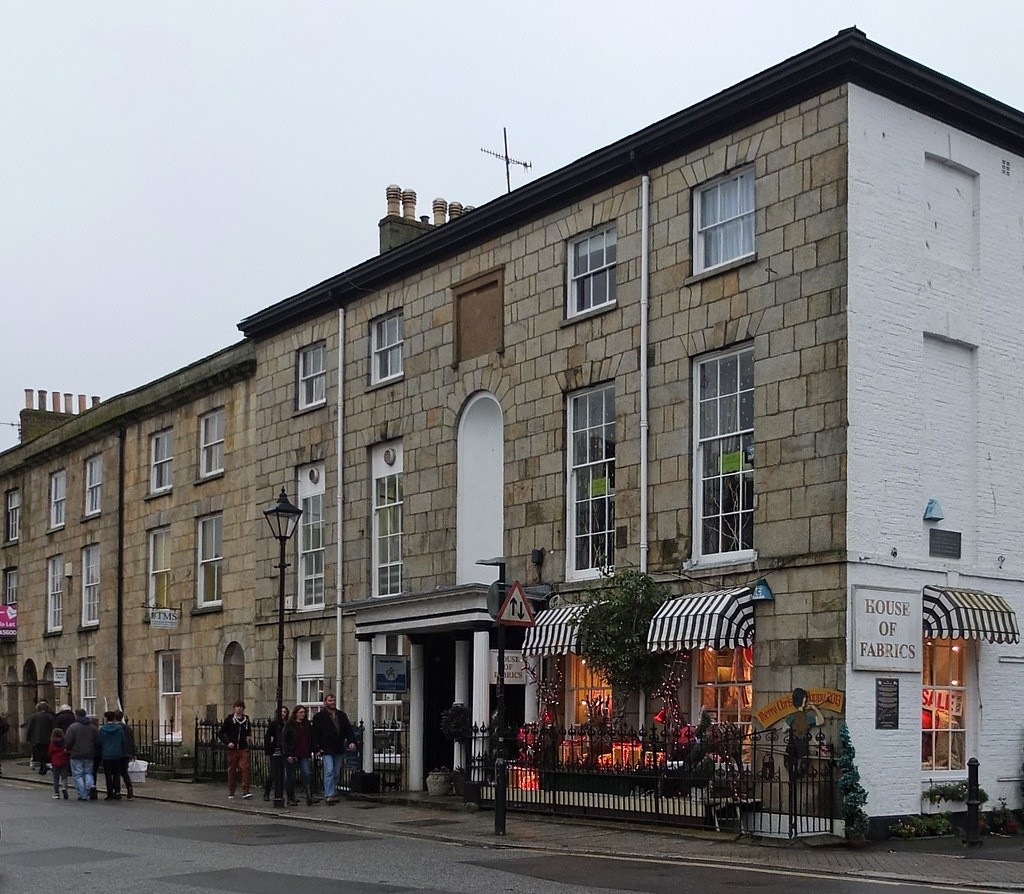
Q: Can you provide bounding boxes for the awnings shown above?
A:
[647,587,755,652]
[520,601,614,659]
[920,584,1021,647]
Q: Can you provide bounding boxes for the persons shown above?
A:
[20,700,136,802]
[218,699,254,799]
[263,695,357,806]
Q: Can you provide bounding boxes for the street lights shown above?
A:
[262,485,303,810]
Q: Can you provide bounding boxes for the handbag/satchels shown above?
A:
[122,756,149,784]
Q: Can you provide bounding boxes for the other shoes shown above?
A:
[103,793,122,801]
[52,794,59,799]
[127,787,133,798]
[77,796,87,800]
[325,795,340,803]
[62,788,68,799]
[242,792,253,798]
[90,786,98,800]
[228,795,235,798]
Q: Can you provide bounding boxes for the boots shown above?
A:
[264,778,273,801]
[286,781,300,803]
[305,784,320,806]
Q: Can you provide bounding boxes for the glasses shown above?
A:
[298,711,306,714]
[282,710,287,713]
